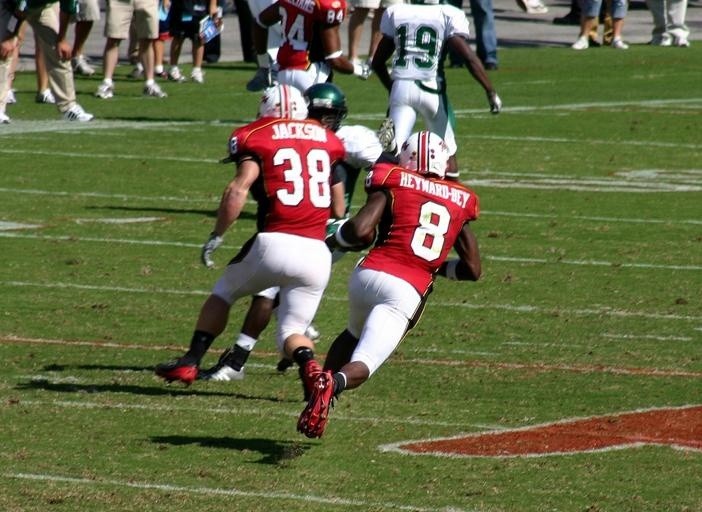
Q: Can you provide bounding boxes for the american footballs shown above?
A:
[349,226,376,251]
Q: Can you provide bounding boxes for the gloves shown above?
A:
[201,230,223,270]
[353,63,373,81]
[256,51,275,69]
[488,91,503,115]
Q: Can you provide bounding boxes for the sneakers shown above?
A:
[68,54,95,76]
[570,32,630,52]
[35,89,56,104]
[154,348,246,385]
[126,63,205,84]
[0,90,16,125]
[375,117,396,152]
[61,104,96,122]
[277,324,339,440]
[347,57,374,65]
[447,58,498,71]
[93,79,114,99]
[143,83,167,99]
[650,31,690,47]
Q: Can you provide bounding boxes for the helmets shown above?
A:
[302,83,349,134]
[258,83,309,122]
[399,130,450,181]
